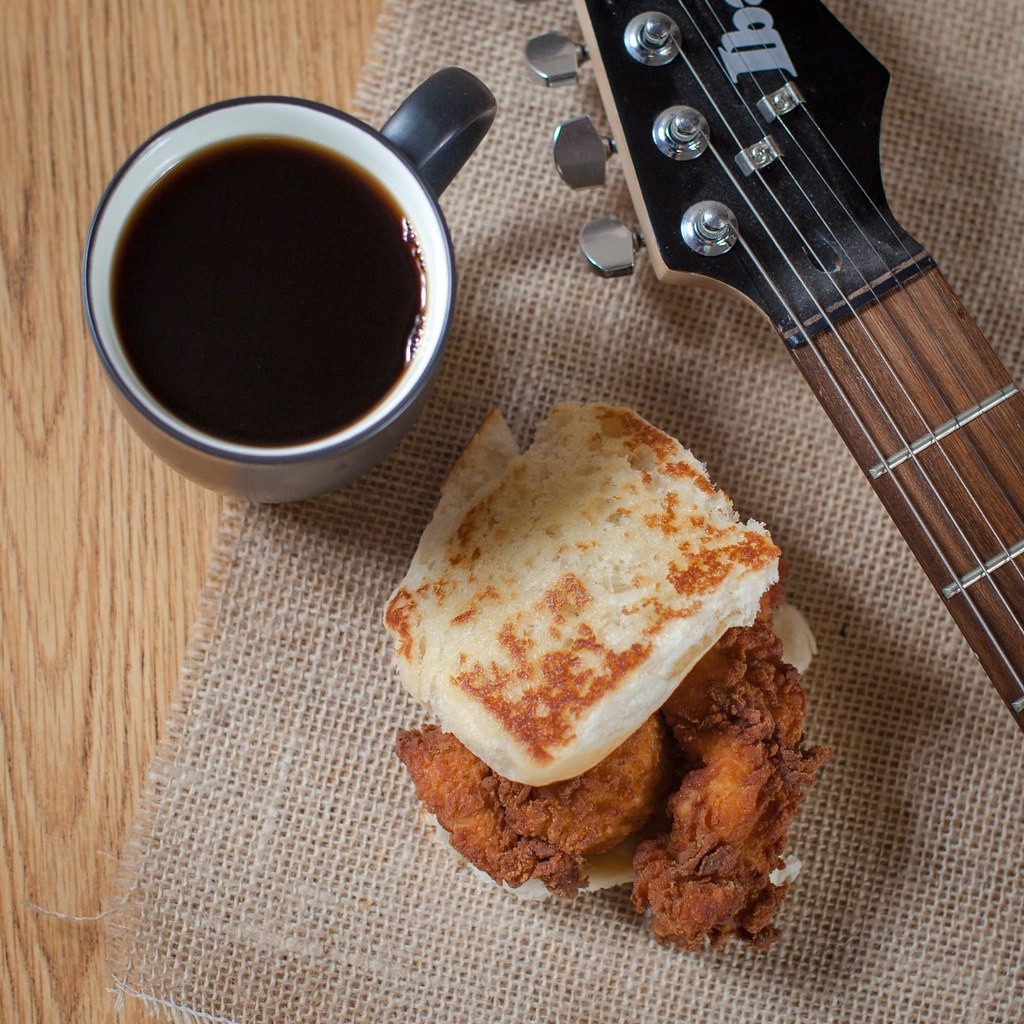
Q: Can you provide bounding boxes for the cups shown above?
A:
[80,65,498,505]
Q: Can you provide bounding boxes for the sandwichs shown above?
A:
[377,397,819,949]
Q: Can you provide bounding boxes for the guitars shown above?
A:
[518,1,1024,736]
